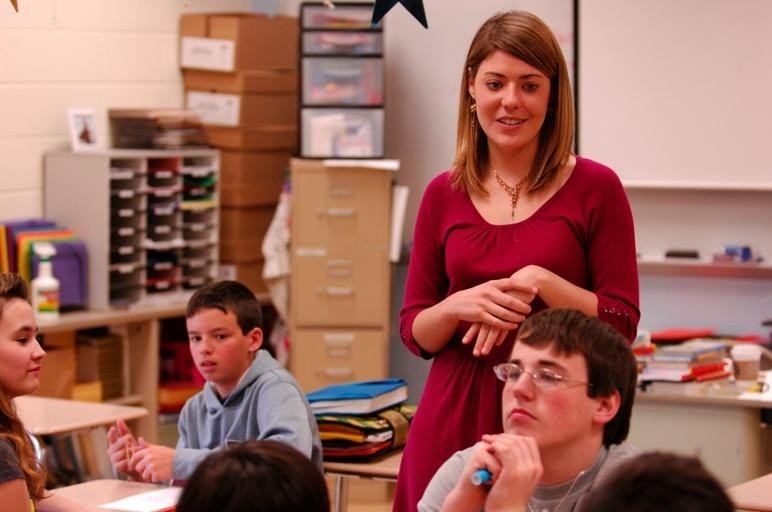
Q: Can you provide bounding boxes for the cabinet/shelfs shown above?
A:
[43,148,221,309]
[289,330,390,395]
[382,2,771,188]
[620,183,772,408]
[287,157,397,329]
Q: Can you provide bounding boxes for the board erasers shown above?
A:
[666,250,699,259]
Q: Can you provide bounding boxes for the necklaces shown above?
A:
[489,162,534,218]
[526,471,584,512]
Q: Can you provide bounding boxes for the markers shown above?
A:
[714,254,742,264]
[471,470,491,485]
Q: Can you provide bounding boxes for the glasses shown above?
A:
[493,360,606,391]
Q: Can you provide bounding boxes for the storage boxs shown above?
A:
[179,12,301,293]
[299,3,385,159]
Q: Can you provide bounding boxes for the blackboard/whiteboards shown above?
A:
[382,0,772,279]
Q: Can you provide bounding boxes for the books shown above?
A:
[0,217,90,308]
[634,323,772,399]
[304,378,417,465]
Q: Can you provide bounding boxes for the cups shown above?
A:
[730,343,763,390]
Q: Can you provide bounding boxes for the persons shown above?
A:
[107,279,324,486]
[393,11,640,512]
[417,308,639,512]
[0,272,132,512]
[174,439,332,512]
[578,450,736,512]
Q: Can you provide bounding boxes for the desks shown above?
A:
[34,479,184,512]
[32,291,272,445]
[322,448,403,512]
[13,396,149,436]
[727,471,772,512]
